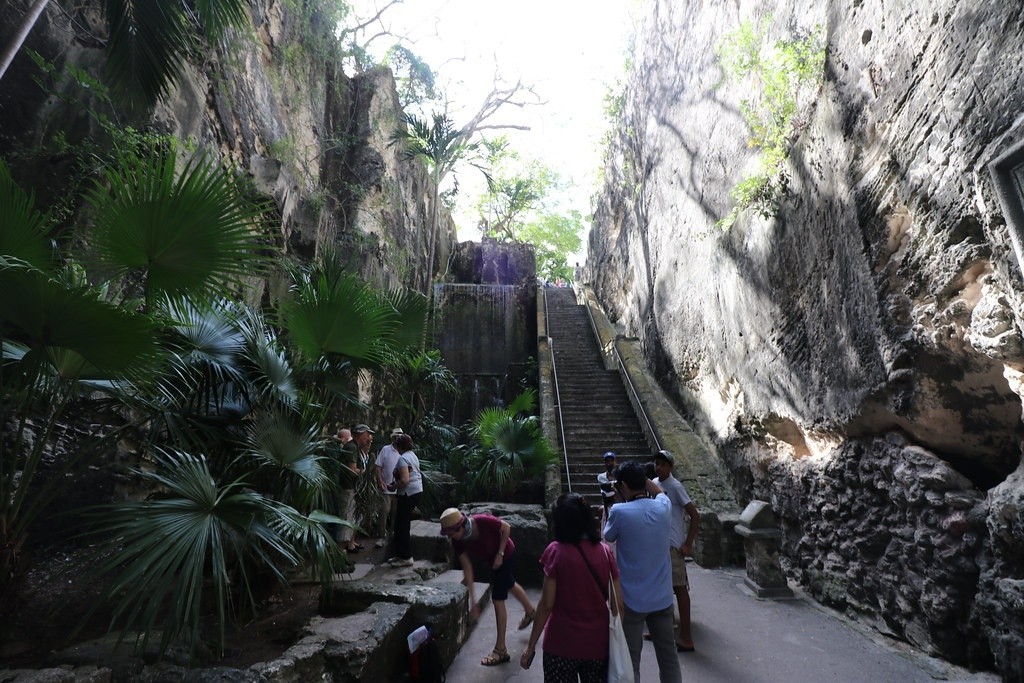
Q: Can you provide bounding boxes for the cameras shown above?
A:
[601,482,618,493]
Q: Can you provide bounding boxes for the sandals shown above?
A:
[518,608,536,629]
[481,647,509,665]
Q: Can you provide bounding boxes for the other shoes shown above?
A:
[375,537,385,548]
[344,545,359,553]
[600,542,634,683]
[676,642,695,653]
[389,556,398,562]
[354,543,364,548]
[391,557,414,567]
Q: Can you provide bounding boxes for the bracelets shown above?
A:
[498,551,504,556]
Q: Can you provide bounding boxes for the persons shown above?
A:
[520,492,625,683]
[643,450,699,652]
[597,452,626,563]
[333,424,376,555]
[375,427,404,549]
[387,435,423,567]
[440,508,534,666]
[574,262,582,270]
[537,274,572,287]
[603,459,682,683]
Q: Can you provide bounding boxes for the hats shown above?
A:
[653,450,674,467]
[440,508,465,534]
[604,452,616,462]
[390,428,404,439]
[352,424,375,434]
